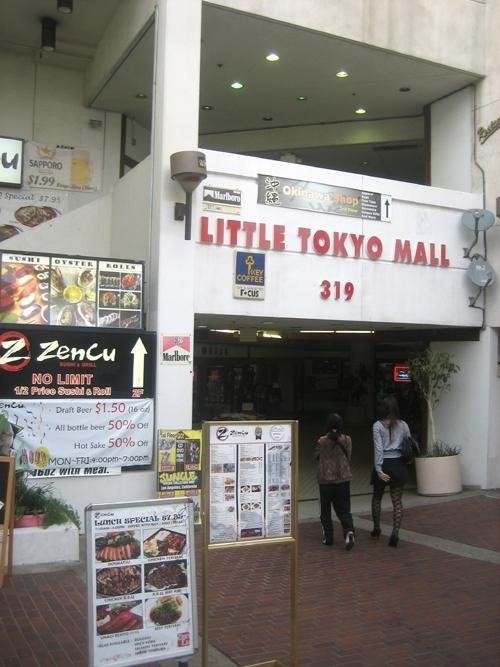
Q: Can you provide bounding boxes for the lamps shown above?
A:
[57,0,74,15]
[41,16,57,52]
[170,150,207,239]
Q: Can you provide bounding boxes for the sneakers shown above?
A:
[345,531,355,551]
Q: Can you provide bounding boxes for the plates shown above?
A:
[41,304,49,323]
[142,528,187,559]
[0,223,22,242]
[36,273,46,280]
[13,203,62,228]
[145,594,191,627]
[241,486,250,493]
[242,504,250,511]
[96,565,142,598]
[253,502,261,511]
[77,302,95,326]
[33,265,44,272]
[41,292,50,302]
[64,285,83,303]
[56,305,77,326]
[93,536,142,563]
[38,282,49,289]
[97,602,144,633]
[20,292,36,307]
[144,562,188,593]
[77,267,97,288]
[50,267,64,290]
[20,304,42,319]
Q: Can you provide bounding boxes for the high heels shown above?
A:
[388,537,399,547]
[370,528,381,540]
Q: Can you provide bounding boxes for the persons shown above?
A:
[314,412,355,551]
[369,396,411,548]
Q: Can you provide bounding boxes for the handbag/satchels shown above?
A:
[402,438,413,462]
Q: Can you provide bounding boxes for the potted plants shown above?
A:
[14,471,81,531]
[405,346,464,496]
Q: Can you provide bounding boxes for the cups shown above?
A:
[70,149,94,186]
